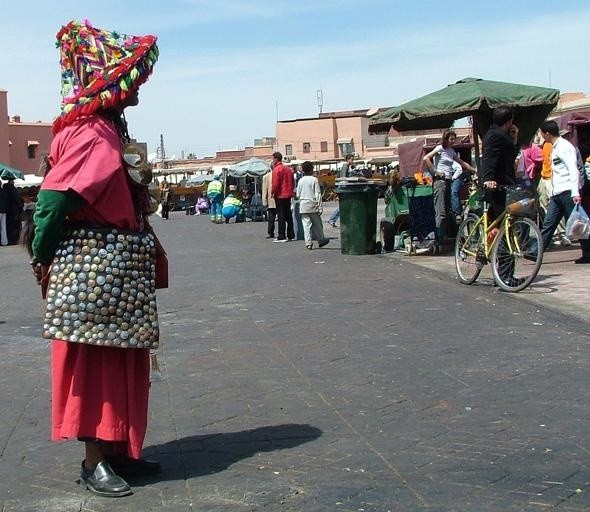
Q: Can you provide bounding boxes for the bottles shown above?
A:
[415,170,432,186]
[507,197,536,213]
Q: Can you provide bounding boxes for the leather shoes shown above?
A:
[493,276,525,287]
[79,451,161,497]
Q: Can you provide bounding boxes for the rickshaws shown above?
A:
[379,178,433,253]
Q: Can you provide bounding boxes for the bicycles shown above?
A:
[454,182,545,293]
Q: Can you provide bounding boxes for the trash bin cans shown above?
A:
[336,183,381,255]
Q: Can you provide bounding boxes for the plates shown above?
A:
[126,165,152,186]
[121,143,145,167]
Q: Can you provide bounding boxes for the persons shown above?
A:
[27,20,169,499]
[422,118,589,265]
[160,183,171,219]
[328,153,355,227]
[2,178,23,245]
[193,151,330,251]
[0,180,9,246]
[481,106,526,288]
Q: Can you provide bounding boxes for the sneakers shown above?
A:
[265,231,297,242]
[305,244,313,250]
[575,256,590,263]
[318,238,329,247]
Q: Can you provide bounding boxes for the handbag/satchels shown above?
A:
[41,225,161,350]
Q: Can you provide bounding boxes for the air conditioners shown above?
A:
[352,152,358,157]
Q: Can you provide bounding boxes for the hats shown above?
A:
[53,17,157,110]
